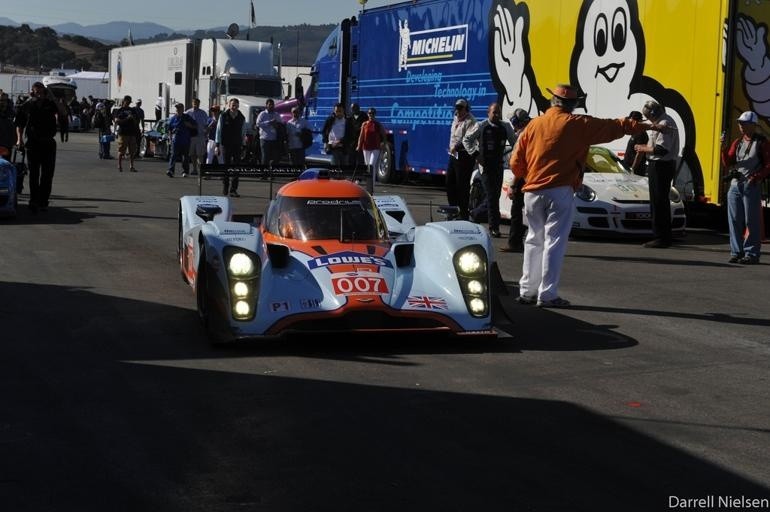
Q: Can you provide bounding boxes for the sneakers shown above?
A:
[642,240,665,249]
[728,256,741,263]
[537,297,569,307]
[489,227,501,237]
[738,257,759,265]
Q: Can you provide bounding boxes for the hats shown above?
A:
[736,110,757,125]
[546,85,587,100]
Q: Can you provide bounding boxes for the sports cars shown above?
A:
[177,168,514,350]
[138,118,261,165]
[0,146,17,218]
[470,146,686,242]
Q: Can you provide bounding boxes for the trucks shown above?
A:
[0,68,109,131]
[107,22,292,158]
[264,0,768,208]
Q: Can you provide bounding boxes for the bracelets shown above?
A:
[629,166,638,171]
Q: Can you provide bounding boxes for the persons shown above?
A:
[635,101,679,249]
[444,98,478,220]
[509,85,677,308]
[463,103,516,237]
[719,111,768,264]
[0,81,386,214]
[501,109,532,253]
[623,111,649,175]
